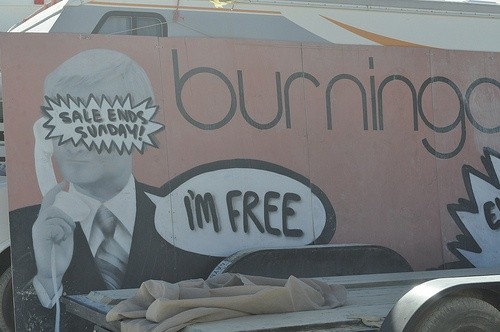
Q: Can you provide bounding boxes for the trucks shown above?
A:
[6,1,500,53]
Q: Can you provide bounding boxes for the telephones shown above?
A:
[33,116,91,223]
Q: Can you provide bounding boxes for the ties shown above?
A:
[95,205,129,290]
